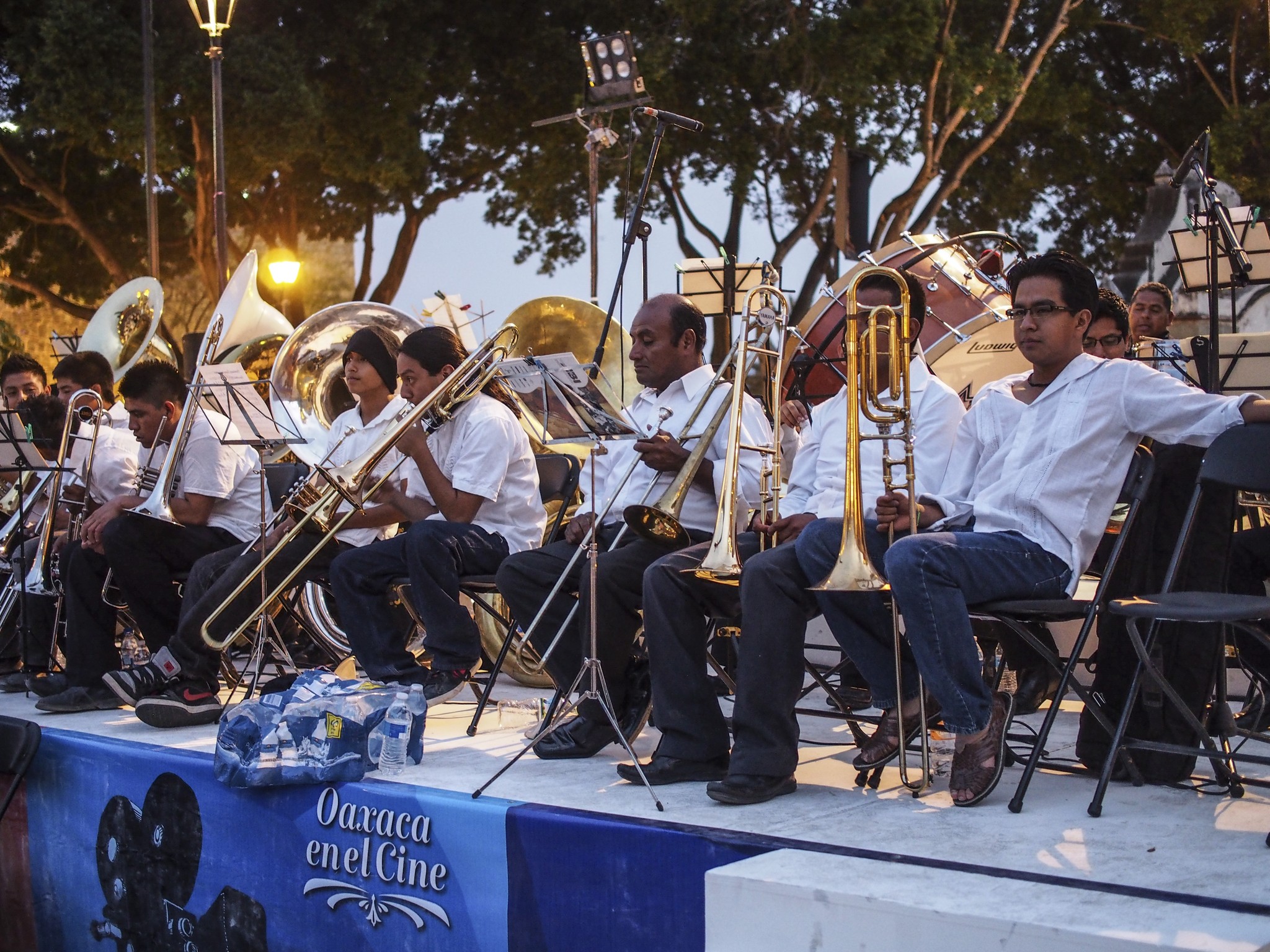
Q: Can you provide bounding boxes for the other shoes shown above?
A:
[26,673,76,697]
[35,683,125,713]
[0,668,32,691]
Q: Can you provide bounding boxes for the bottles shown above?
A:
[972,636,984,676]
[310,718,327,754]
[496,697,578,729]
[377,692,413,777]
[132,640,151,669]
[257,721,297,769]
[407,683,428,765]
[120,627,139,669]
[995,642,1018,696]
[341,682,366,783]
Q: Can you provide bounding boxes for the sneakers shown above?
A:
[135,685,222,728]
[101,645,182,706]
[422,658,482,709]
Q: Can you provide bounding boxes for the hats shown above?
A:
[342,326,402,395]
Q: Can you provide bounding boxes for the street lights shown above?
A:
[192,0,240,296]
[572,25,653,304]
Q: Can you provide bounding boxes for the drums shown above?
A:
[778,228,1034,434]
[1132,339,1193,385]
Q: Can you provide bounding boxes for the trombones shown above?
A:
[0,408,114,636]
[679,284,788,587]
[6,388,102,673]
[202,323,519,652]
[805,264,928,799]
[514,317,775,675]
[101,315,224,609]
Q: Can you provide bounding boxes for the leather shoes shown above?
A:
[824,684,872,710]
[616,751,731,784]
[617,690,653,749]
[532,706,641,759]
[1012,668,1068,715]
[1230,690,1270,732]
[706,772,797,804]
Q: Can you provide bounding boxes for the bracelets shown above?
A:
[915,502,920,526]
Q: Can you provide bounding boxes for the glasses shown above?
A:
[1082,333,1124,349]
[1005,305,1079,322]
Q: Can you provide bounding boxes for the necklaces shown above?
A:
[1028,373,1050,387]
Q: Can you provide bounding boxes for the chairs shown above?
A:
[239,421,1270,850]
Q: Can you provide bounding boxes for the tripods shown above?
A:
[472,354,665,813]
[0,396,77,696]
[185,372,315,722]
[1205,626,1270,799]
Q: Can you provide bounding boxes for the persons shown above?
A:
[617,267,966,805]
[997,287,1130,716]
[79,363,273,660]
[494,293,773,759]
[327,327,547,707]
[101,326,415,729]
[0,350,132,697]
[13,395,169,713]
[794,250,1270,807]
[1122,282,1175,360]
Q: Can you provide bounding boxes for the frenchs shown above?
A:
[476,297,646,689]
[270,301,428,680]
[196,249,299,464]
[0,276,179,519]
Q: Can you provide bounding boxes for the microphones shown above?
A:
[636,107,704,132]
[1170,130,1208,188]
[762,260,780,283]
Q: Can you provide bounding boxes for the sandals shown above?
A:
[948,690,1014,807]
[853,696,942,771]
